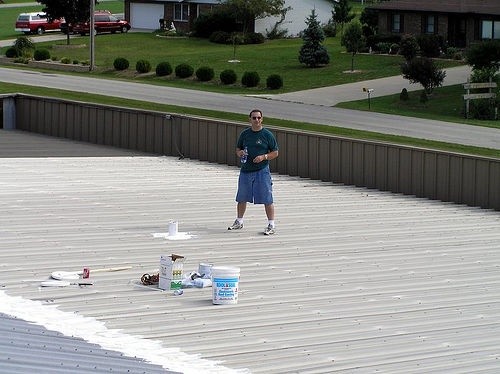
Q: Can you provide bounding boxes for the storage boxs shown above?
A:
[158,254,185,290]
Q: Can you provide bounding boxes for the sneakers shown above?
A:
[228,219,244,230]
[263,223,277,235]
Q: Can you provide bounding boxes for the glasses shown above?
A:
[250,117,262,120]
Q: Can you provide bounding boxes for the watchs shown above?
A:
[264,154,268,160]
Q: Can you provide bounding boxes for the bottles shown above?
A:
[241,147,247,163]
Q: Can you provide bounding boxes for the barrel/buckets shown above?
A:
[198,263,213,279]
[210,266,240,305]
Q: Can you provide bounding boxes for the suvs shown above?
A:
[14,12,65,36]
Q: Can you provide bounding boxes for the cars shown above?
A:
[70,10,131,36]
[59,18,73,34]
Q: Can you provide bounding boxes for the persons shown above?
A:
[228,109,279,235]
